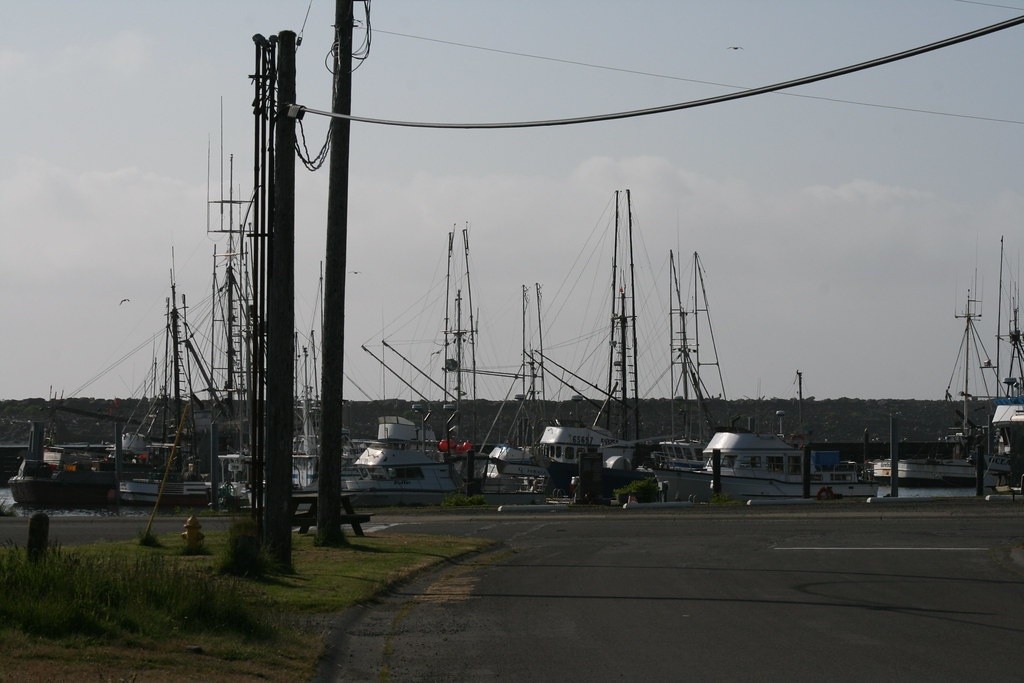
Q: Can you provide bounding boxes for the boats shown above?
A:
[7,184,743,514]
[651,431,881,502]
[863,422,981,488]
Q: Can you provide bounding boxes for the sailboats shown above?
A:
[941,232,1024,488]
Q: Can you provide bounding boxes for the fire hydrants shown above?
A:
[181,515,204,552]
